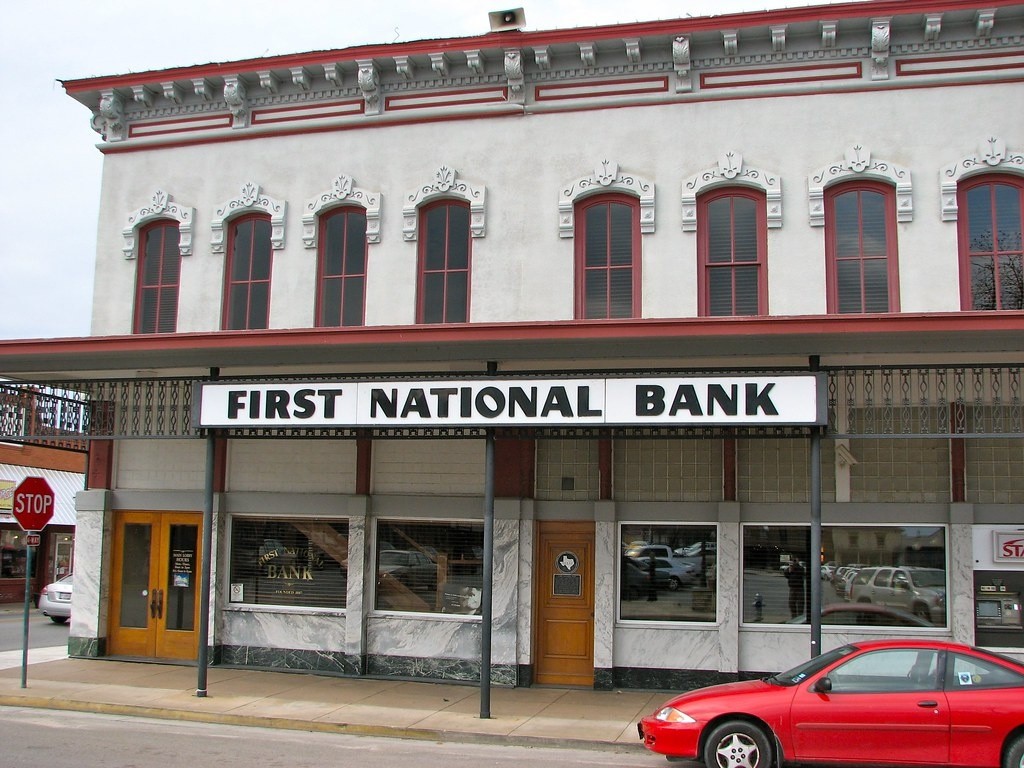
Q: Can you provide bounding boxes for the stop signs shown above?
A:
[12,476,55,532]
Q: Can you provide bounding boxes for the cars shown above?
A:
[232,538,299,579]
[637,638,1024,768]
[778,559,1024,629]
[377,548,440,593]
[618,536,719,599]
[37,572,73,624]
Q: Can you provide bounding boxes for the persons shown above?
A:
[645,553,658,601]
[785,555,804,618]
[451,574,483,616]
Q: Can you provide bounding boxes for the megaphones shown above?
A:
[489,7,526,33]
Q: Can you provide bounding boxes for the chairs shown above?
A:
[907,663,936,688]
